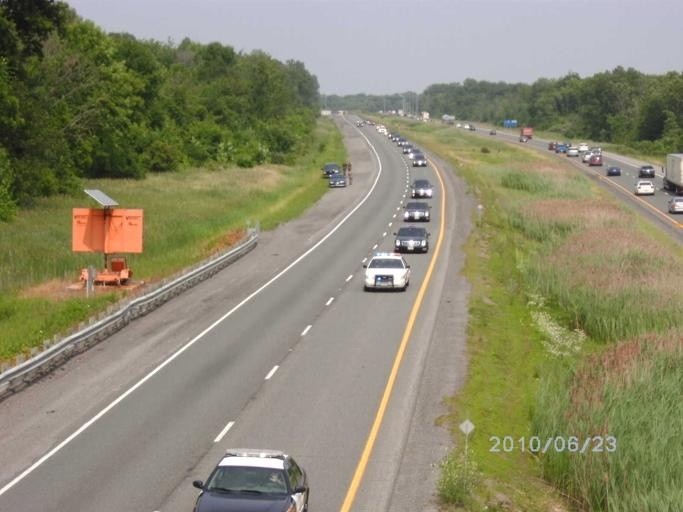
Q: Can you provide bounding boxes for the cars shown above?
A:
[363,179,434,290]
[607,166,621,175]
[634,165,655,195]
[668,196,683,214]
[193,448,309,512]
[356,120,427,167]
[321,162,346,187]
[519,137,528,142]
[548,140,603,166]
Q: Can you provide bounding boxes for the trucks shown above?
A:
[661,153,683,195]
[520,127,534,139]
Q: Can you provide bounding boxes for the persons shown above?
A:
[342,160,353,185]
